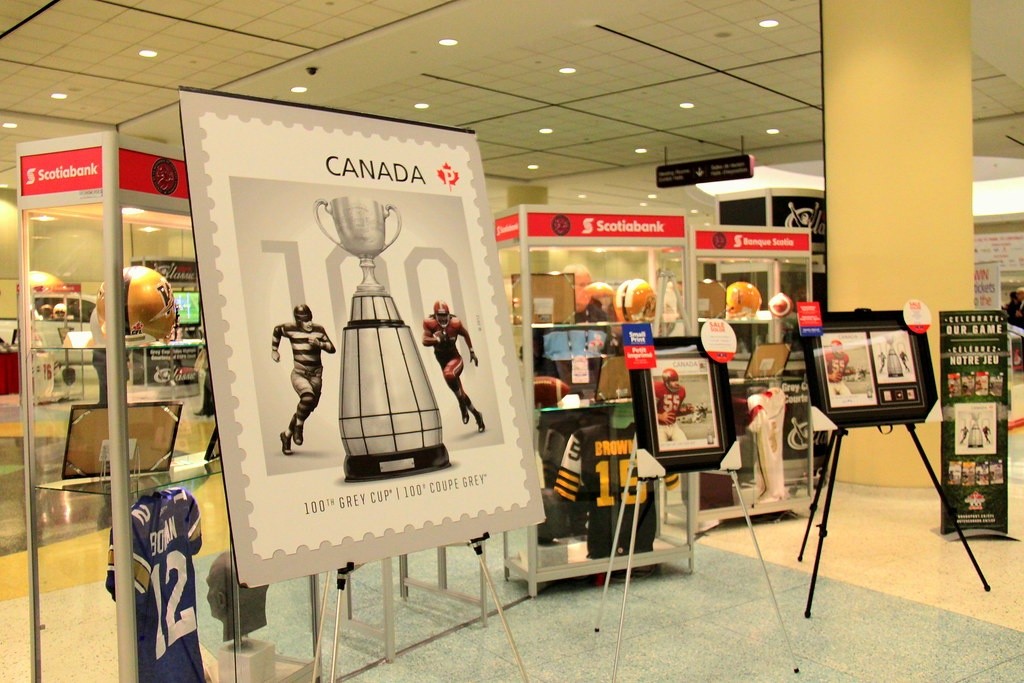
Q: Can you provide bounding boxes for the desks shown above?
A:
[0,350,49,396]
[129,339,204,386]
[723,376,836,482]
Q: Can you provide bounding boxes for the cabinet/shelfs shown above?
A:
[15,132,322,683]
[17,284,85,406]
[493,203,814,600]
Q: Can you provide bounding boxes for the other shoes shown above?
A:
[280,433,292,454]
[294,417,303,444]
[460,405,469,424]
[475,413,484,432]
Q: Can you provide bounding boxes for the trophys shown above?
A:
[884,335,904,377]
[968,412,984,448]
[313,196,454,481]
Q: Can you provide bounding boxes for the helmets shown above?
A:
[90,266,178,348]
[663,368,680,393]
[831,340,843,358]
[434,300,450,328]
[726,282,762,319]
[615,279,655,323]
[53,303,66,320]
[293,304,313,321]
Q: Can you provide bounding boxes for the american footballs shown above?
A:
[533,376,571,410]
[769,292,794,318]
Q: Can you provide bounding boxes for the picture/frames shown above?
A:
[744,343,792,378]
[594,356,631,401]
[627,336,742,477]
[799,310,945,433]
[676,280,728,319]
[61,400,183,481]
[511,273,577,325]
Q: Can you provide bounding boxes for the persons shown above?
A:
[421,301,486,434]
[1014,287,1024,374]
[653,368,695,444]
[878,352,886,373]
[899,351,911,372]
[825,340,856,397]
[982,426,991,444]
[549,265,617,406]
[270,302,337,456]
[1007,291,1021,327]
[207,549,268,643]
[959,427,969,444]
